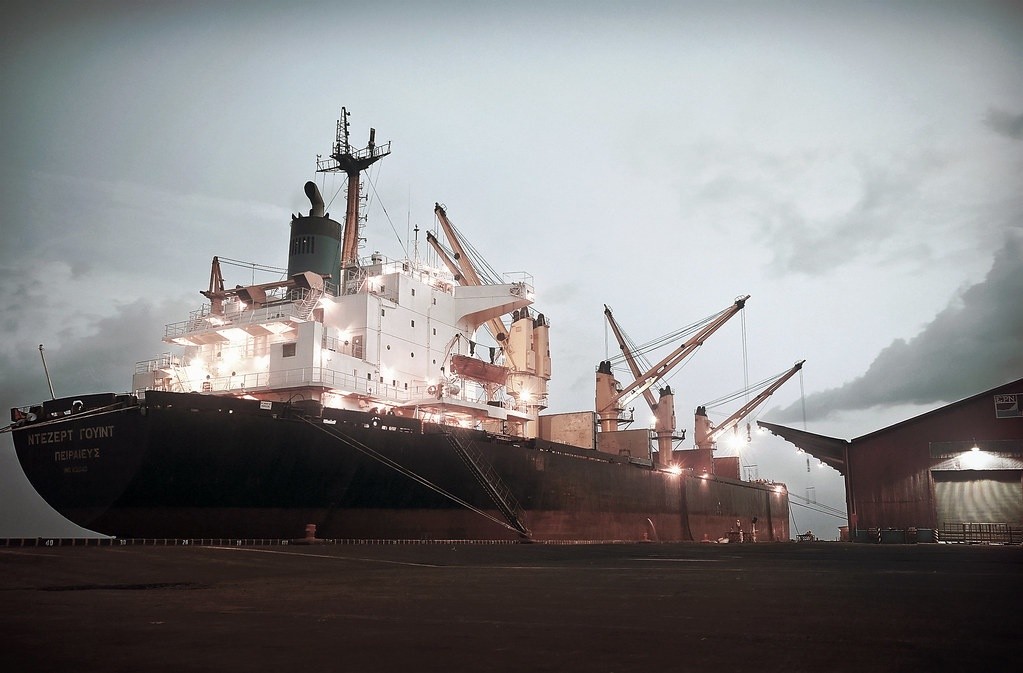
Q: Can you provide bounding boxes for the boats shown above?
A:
[0,107,806,540]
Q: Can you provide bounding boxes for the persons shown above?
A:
[735,519,741,528]
[738,530,744,541]
